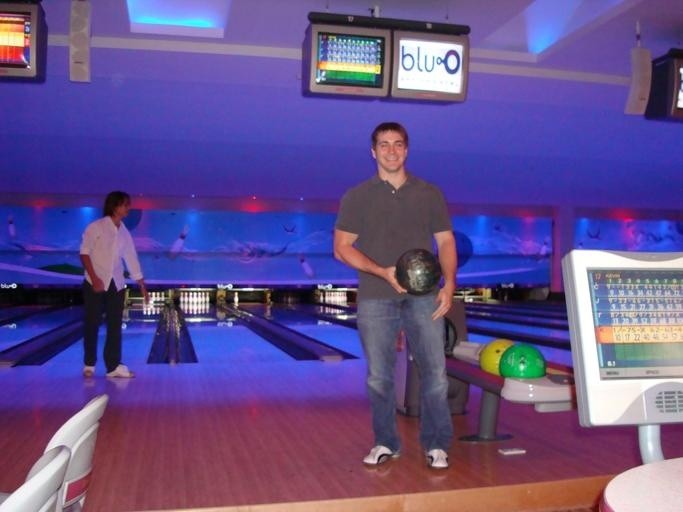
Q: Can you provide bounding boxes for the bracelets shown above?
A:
[138,284,145,288]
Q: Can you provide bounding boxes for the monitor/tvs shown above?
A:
[0,1,46,83]
[392,30,470,105]
[642,52,683,120]
[301,24,391,102]
[561,248,683,428]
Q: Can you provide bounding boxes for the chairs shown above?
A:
[46,393,109,511]
[0,444,71,512]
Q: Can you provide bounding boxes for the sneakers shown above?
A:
[425,448,449,469]
[361,444,400,466]
[105,362,137,379]
[82,364,96,379]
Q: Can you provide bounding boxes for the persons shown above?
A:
[79,191,150,379]
[333,123,458,469]
